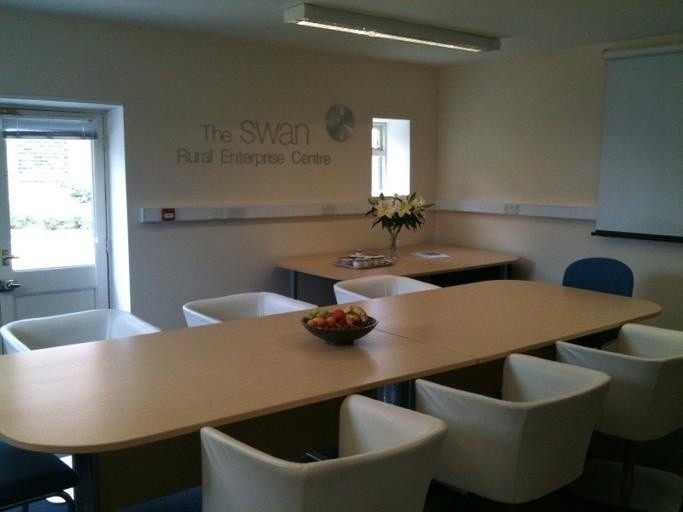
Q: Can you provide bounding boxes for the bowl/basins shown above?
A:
[300,315,379,347]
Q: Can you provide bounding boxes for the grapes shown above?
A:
[304,306,346,331]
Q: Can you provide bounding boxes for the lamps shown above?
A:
[282,3,499,52]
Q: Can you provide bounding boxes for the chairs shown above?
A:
[183,292,318,327]
[334,275,441,304]
[563,257,633,349]
[0,309,161,353]
[0,449,78,512]
[416,354,612,512]
[200,395,448,512]
[556,323,683,512]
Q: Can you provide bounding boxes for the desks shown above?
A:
[276,244,520,299]
[0,280,662,512]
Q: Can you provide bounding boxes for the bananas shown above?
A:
[340,304,367,328]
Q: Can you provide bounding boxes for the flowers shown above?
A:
[365,192,435,251]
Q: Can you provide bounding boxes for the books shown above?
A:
[334,255,393,268]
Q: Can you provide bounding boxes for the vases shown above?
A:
[380,226,403,259]
